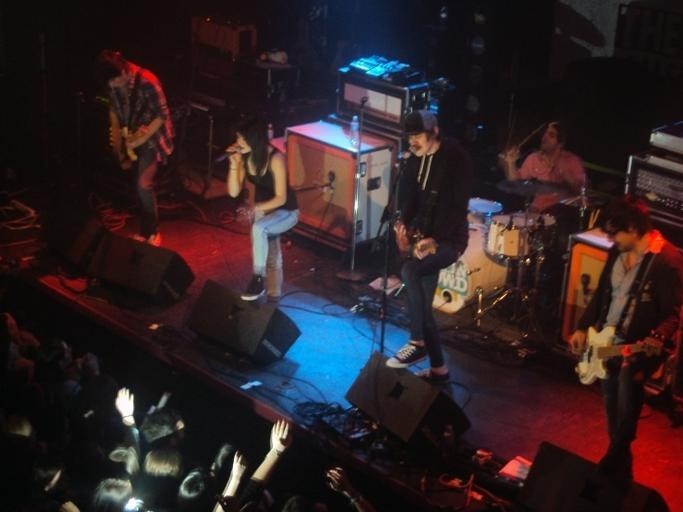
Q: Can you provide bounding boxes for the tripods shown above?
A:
[515,255,546,340]
[471,264,538,334]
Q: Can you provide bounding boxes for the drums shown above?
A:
[485,214,560,265]
[466,198,501,224]
[432,213,511,315]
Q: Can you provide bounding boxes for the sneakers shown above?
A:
[415,370,450,384]
[241,276,265,301]
[133,232,161,245]
[386,341,427,369]
[268,297,280,303]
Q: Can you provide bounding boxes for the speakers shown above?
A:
[79,229,196,307]
[184,278,302,370]
[559,226,615,345]
[345,350,471,456]
[283,120,398,244]
[515,440,669,512]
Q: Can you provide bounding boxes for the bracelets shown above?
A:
[228,166,240,170]
[650,329,666,343]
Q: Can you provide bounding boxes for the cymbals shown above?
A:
[494,180,570,195]
[562,192,613,209]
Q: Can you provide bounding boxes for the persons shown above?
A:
[567,194,683,478]
[96,35,173,247]
[0,304,377,512]
[506,121,586,214]
[226,119,299,302]
[385,110,469,389]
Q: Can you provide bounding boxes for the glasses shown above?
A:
[607,229,620,237]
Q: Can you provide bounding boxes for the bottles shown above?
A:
[349,115,359,146]
[443,425,455,444]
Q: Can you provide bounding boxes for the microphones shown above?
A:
[215,146,241,163]
[395,145,419,168]
[325,170,336,188]
[581,274,591,294]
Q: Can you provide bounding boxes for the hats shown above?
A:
[398,110,437,135]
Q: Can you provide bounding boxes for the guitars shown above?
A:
[575,326,662,386]
[373,223,437,270]
[112,72,140,171]
